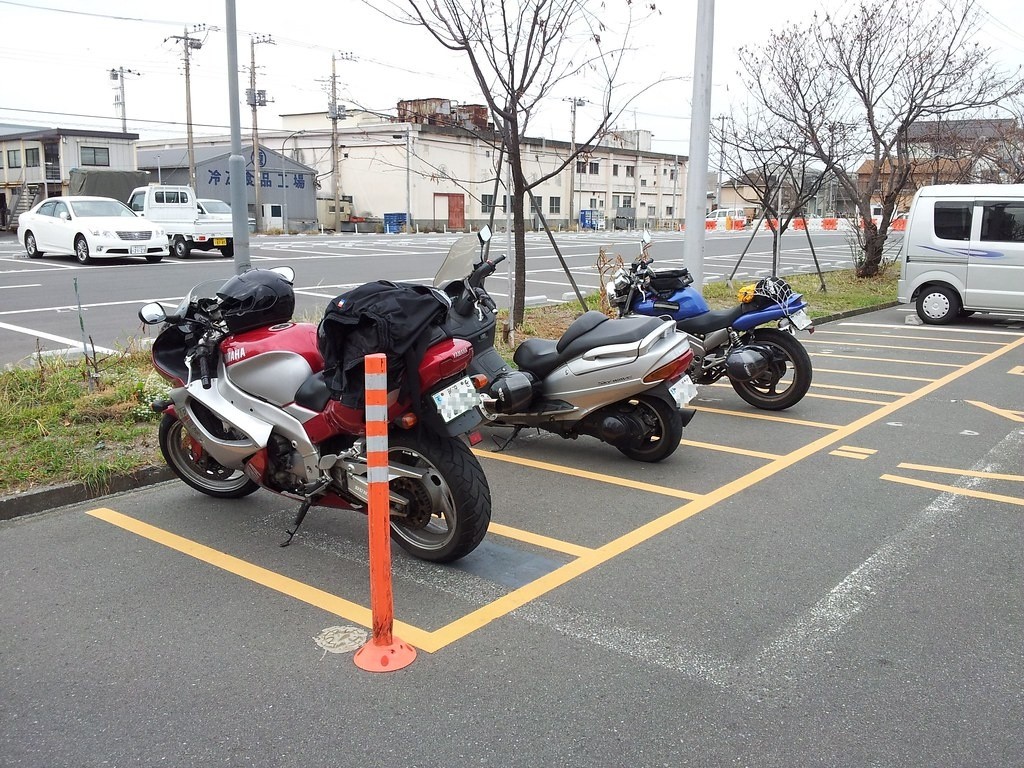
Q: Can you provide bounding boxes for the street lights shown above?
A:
[280,130,306,234]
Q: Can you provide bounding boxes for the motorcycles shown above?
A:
[606,229,817,412]
[136,265,501,566]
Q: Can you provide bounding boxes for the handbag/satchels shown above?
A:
[651,269,692,291]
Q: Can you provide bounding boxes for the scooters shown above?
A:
[418,225,697,465]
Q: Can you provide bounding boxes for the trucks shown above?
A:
[122,184,234,259]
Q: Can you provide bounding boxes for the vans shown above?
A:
[705,208,747,228]
[894,184,1024,325]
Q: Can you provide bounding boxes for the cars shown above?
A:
[195,198,232,233]
[17,196,171,263]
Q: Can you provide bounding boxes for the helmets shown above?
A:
[216,268,295,333]
